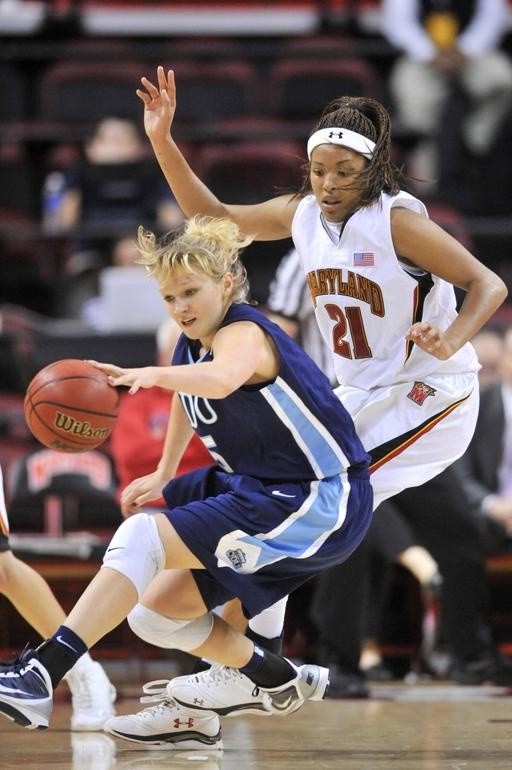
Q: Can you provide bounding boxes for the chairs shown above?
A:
[0,40,512,667]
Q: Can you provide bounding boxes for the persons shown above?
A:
[0,0,510,703]
[1,217,377,735]
[100,59,509,758]
[1,468,119,733]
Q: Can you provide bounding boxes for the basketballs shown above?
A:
[24,360,118,452]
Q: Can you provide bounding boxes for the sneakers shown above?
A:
[0,648,52,729]
[108,661,367,752]
[369,649,511,685]
[70,658,119,732]
